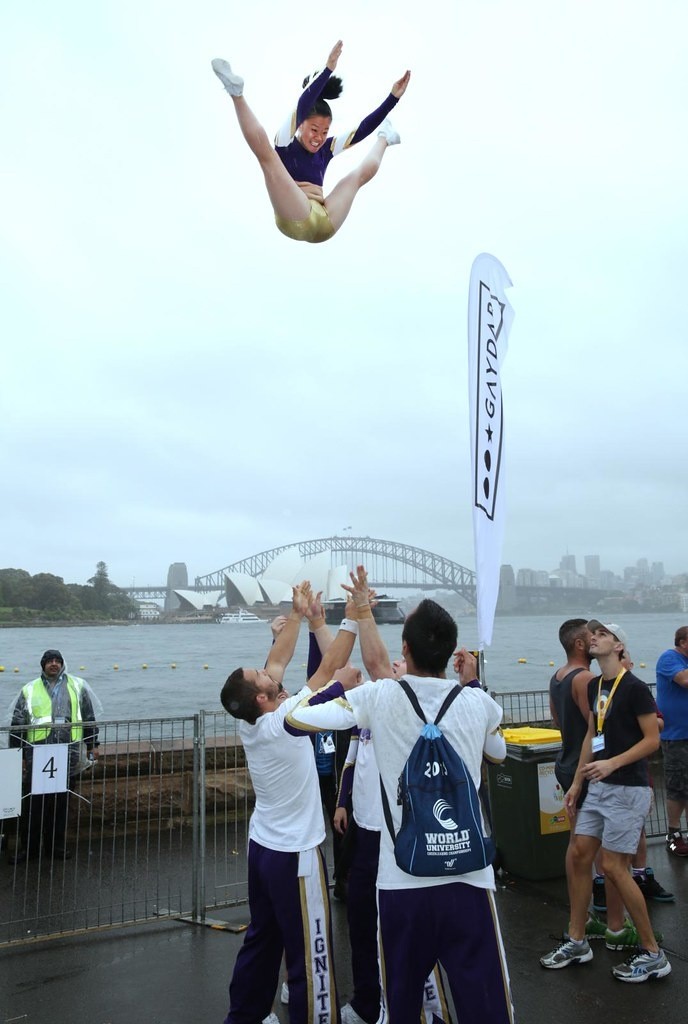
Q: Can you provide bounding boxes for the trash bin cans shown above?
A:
[486,726,572,882]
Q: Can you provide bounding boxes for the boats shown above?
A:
[216,608,270,623]
[321,593,405,624]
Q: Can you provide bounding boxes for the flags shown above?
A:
[471,253,515,646]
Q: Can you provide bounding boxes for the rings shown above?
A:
[590,775,594,779]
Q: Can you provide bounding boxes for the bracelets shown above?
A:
[463,679,480,688]
[356,603,369,608]
[339,619,359,635]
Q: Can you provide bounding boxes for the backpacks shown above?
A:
[379,679,496,877]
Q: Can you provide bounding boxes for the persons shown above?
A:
[540,619,672,983]
[211,39,411,243]
[9,650,102,861]
[550,619,664,951]
[220,563,457,1024]
[657,626,688,857]
[592,647,674,914]
[283,598,516,1024]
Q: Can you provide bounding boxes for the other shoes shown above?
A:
[45,843,72,862]
[280,982,290,1004]
[262,1013,280,1024]
[10,849,33,864]
[341,1002,367,1024]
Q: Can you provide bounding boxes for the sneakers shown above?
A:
[605,922,665,950]
[633,867,674,902]
[592,876,608,909]
[611,947,672,982]
[538,936,593,970]
[563,914,607,941]
[664,833,688,857]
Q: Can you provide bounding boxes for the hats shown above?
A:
[586,618,628,650]
